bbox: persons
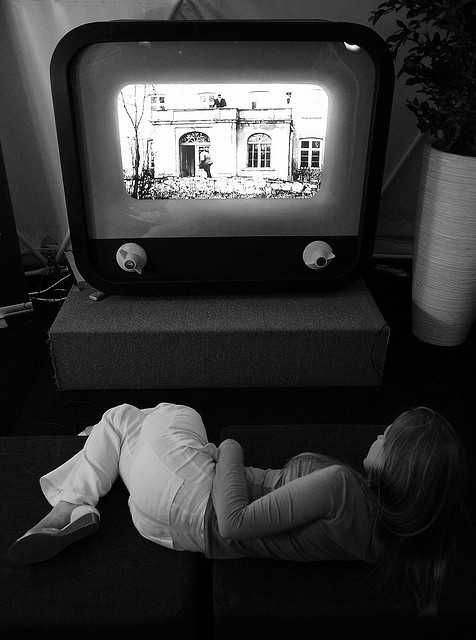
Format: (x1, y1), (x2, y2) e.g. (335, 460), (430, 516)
(6, 400), (459, 565)
(212, 94), (226, 107)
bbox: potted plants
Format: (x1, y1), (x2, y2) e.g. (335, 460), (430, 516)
(368, 0), (476, 346)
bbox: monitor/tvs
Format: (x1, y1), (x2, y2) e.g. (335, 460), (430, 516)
(48, 17), (397, 298)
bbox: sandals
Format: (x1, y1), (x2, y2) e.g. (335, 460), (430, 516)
(7, 505), (101, 564)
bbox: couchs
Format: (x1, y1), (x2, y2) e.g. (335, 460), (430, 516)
(0, 425), (476, 638)
